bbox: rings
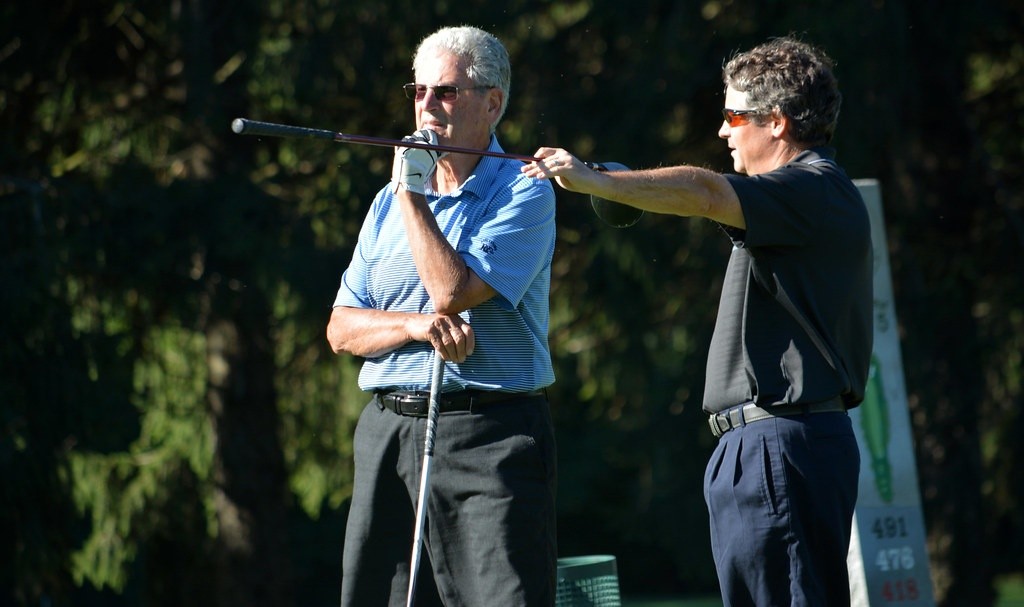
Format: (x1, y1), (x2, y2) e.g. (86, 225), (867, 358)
(553, 160), (559, 166)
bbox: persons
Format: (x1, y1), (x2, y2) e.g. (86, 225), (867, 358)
(327, 24), (560, 607)
(520, 38), (874, 607)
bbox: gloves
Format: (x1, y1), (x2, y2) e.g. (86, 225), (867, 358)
(390, 129), (448, 195)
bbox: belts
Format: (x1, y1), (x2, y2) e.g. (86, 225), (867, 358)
(372, 385), (544, 418)
(709, 394), (845, 436)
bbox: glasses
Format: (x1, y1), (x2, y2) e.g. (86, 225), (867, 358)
(722, 106), (772, 125)
(403, 82), (496, 103)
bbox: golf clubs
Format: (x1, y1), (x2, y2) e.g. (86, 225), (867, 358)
(405, 346), (445, 607)
(229, 118), (643, 229)
